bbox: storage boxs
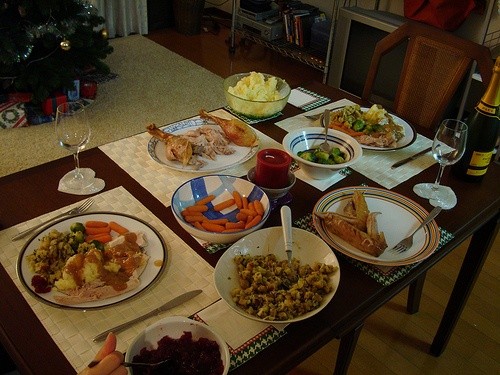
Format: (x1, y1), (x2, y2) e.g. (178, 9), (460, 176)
(311, 21), (337, 53)
(0, 76), (97, 130)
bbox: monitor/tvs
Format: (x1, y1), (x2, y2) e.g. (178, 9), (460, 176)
(328, 6), (410, 111)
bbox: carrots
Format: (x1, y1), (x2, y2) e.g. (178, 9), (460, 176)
(85, 220), (129, 243)
(182, 191), (263, 233)
(328, 120), (366, 137)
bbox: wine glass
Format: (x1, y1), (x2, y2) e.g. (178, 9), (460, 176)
(420, 118), (468, 202)
(55, 102), (94, 190)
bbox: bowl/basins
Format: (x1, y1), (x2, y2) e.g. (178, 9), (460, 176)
(247, 167), (296, 199)
(222, 73), (292, 118)
(123, 316), (230, 375)
(282, 127), (363, 181)
(212, 228), (341, 325)
(170, 174), (271, 244)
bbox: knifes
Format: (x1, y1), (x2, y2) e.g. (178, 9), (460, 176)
(91, 289), (203, 342)
(391, 143), (440, 169)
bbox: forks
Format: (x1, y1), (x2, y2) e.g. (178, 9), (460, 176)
(302, 105), (345, 120)
(11, 196), (95, 242)
(392, 206), (442, 255)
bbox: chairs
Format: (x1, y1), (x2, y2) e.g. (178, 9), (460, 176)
(362, 20), (493, 315)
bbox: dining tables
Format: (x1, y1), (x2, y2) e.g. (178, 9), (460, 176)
(0, 80), (500, 375)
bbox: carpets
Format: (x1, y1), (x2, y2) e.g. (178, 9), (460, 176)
(0, 34), (226, 178)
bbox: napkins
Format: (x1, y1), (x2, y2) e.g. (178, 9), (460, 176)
(197, 299), (272, 350)
(287, 88), (319, 108)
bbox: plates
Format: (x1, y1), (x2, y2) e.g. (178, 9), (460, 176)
(320, 108), (418, 152)
(16, 212), (168, 309)
(147, 117), (261, 173)
(312, 187), (440, 267)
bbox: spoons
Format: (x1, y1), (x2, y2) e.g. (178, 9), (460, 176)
(319, 109), (330, 153)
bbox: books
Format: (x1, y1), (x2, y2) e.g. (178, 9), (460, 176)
(283, 9), (314, 48)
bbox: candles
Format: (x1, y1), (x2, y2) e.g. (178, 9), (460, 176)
(255, 149), (292, 189)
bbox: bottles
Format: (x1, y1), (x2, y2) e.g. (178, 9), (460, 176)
(450, 54), (500, 186)
